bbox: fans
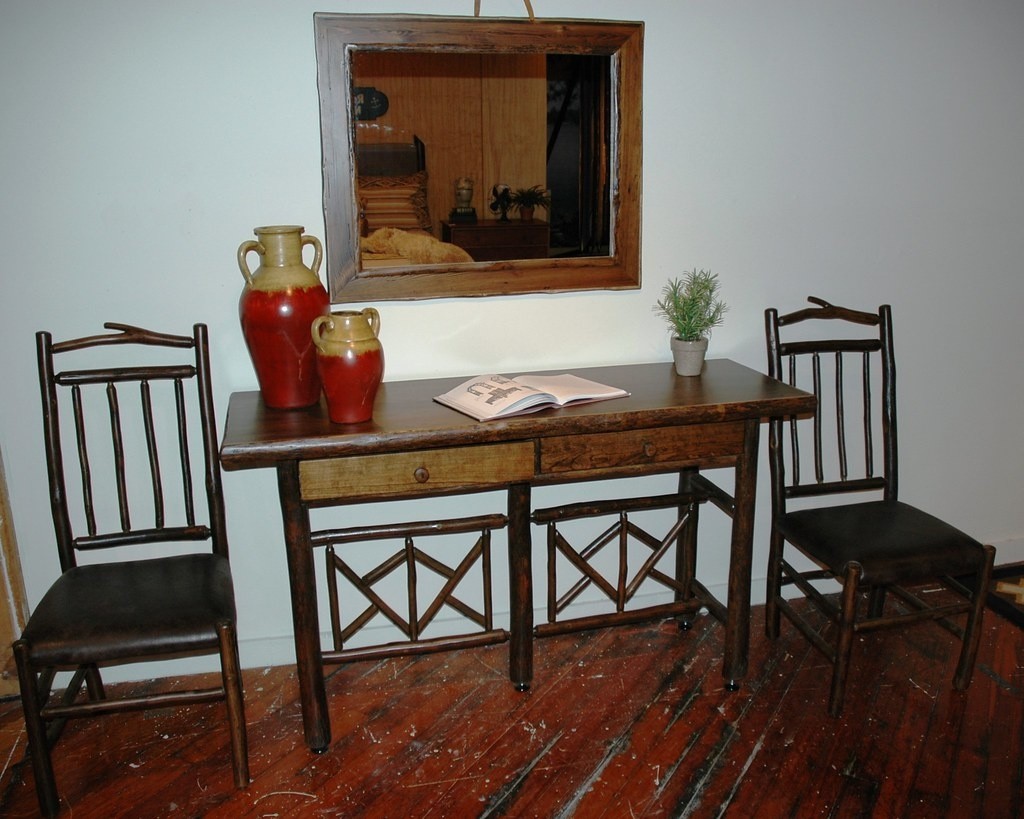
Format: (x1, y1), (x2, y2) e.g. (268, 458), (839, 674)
(486, 183), (513, 223)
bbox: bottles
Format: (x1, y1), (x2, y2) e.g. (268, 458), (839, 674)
(311, 308), (383, 423)
(237, 225), (328, 408)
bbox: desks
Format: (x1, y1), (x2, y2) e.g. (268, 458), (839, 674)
(220, 359), (817, 754)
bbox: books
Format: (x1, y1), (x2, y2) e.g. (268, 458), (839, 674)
(432, 373), (630, 423)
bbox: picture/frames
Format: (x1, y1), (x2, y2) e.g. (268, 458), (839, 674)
(314, 13), (646, 305)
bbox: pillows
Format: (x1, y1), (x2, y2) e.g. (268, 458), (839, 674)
(354, 172), (432, 233)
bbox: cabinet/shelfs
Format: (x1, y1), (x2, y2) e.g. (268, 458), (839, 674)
(441, 218), (549, 264)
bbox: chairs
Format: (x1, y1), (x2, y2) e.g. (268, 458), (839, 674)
(764, 296), (996, 719)
(12, 322), (250, 819)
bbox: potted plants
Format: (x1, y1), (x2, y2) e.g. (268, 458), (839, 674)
(652, 268), (732, 377)
(509, 184), (551, 222)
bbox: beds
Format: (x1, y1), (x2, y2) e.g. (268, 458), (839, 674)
(352, 134), (476, 271)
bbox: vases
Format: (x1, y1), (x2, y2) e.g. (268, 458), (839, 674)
(237, 225), (330, 410)
(311, 307), (385, 424)
